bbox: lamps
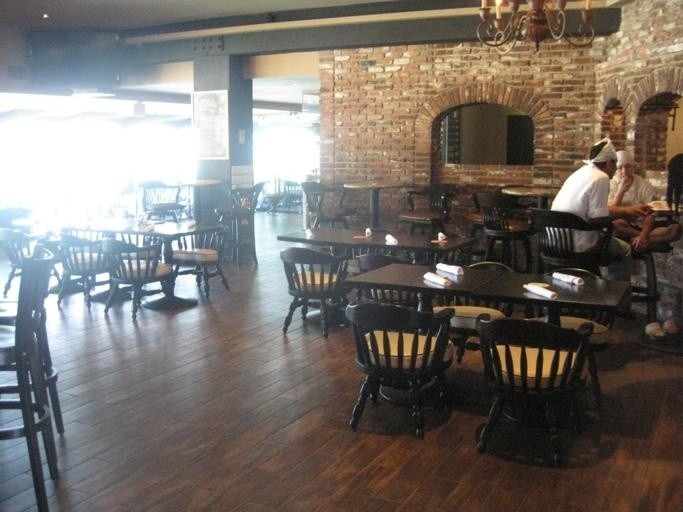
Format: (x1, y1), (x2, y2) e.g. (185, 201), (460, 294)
(473, 1), (598, 56)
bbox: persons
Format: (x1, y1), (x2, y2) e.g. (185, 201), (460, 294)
(667, 152), (682, 215)
(546, 138), (637, 320)
(606, 150), (682, 252)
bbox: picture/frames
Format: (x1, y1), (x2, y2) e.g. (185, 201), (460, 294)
(190, 89), (230, 162)
